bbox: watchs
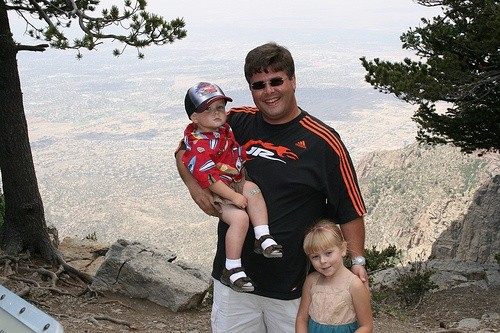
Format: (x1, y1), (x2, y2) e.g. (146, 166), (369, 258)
(350, 256), (365, 265)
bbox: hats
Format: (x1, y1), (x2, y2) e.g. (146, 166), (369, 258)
(184, 81), (232, 118)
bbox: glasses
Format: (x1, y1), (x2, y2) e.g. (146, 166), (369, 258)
(250, 75), (290, 89)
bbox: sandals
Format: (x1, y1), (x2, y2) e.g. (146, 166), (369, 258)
(220, 266), (255, 292)
(253, 234), (283, 258)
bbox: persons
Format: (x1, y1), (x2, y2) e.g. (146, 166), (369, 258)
(176, 81), (283, 292)
(294, 222), (375, 333)
(206, 43), (369, 333)
